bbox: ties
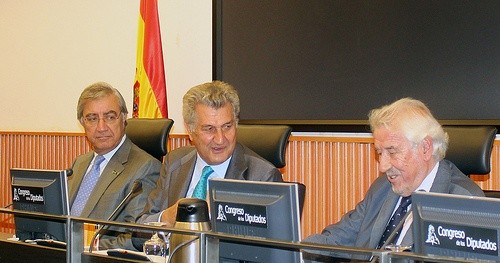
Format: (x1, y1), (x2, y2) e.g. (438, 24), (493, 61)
(192, 166), (214, 201)
(70, 156), (105, 217)
(368, 196), (412, 263)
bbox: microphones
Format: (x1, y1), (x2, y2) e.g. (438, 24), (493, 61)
(88, 180), (142, 254)
(4, 168), (73, 208)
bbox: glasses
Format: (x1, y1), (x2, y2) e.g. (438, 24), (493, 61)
(82, 110), (122, 125)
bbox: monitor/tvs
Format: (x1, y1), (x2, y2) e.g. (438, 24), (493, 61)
(9, 168), (70, 244)
(411, 190), (500, 263)
(208, 179), (303, 263)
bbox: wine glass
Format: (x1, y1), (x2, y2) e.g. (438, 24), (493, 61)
(385, 245), (412, 252)
(143, 221), (167, 263)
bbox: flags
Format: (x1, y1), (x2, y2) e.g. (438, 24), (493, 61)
(135, 0), (169, 120)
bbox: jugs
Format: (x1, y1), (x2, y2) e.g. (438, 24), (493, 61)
(169, 197), (212, 263)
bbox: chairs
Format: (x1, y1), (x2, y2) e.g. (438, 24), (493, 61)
(125, 118), (174, 164)
(442, 125), (500, 198)
(236, 125), (306, 221)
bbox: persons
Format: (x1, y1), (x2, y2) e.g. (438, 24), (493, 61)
(131, 81), (284, 253)
(301, 98), (487, 263)
(64, 81), (160, 250)
